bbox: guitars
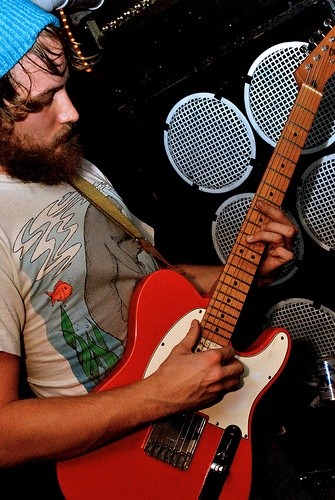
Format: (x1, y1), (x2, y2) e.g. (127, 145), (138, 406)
(55, 17), (335, 500)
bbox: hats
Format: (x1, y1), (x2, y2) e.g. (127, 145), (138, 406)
(0, 0), (62, 78)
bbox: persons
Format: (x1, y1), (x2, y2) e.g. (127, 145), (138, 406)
(0, 0), (300, 469)
